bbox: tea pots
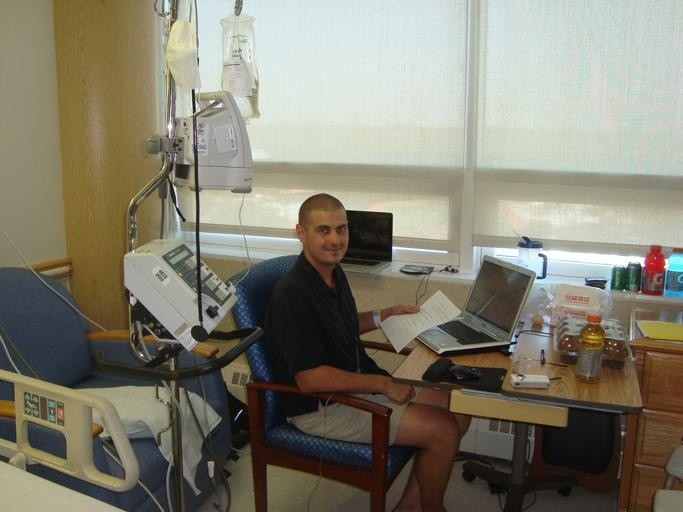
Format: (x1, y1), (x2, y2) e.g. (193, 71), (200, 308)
(515, 236), (547, 281)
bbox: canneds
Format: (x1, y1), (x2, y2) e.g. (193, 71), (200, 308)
(626, 263), (641, 292)
(611, 265), (625, 292)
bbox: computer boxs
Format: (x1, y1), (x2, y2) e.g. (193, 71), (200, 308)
(457, 415), (537, 461)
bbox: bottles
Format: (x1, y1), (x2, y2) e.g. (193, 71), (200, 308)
(665, 247), (683, 298)
(641, 245), (665, 295)
(575, 311), (605, 386)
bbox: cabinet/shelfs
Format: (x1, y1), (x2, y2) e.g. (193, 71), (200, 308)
(616, 334), (682, 511)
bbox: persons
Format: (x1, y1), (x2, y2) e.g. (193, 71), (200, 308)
(262, 193), (471, 511)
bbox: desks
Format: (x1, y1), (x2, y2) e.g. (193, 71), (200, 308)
(390, 320), (644, 512)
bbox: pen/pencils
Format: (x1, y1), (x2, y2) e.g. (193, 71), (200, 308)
(541, 349), (545, 365)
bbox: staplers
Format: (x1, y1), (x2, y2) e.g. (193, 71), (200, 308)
(585, 277), (608, 289)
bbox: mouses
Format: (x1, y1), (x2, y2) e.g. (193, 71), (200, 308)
(450, 364), (481, 382)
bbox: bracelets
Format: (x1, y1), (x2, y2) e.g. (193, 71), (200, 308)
(371, 308), (384, 330)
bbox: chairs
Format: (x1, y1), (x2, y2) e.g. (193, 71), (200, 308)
(0, 266), (235, 511)
(224, 253), (418, 512)
(650, 443), (682, 512)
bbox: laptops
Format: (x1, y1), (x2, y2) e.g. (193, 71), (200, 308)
(339, 209), (393, 274)
(414, 255), (536, 356)
